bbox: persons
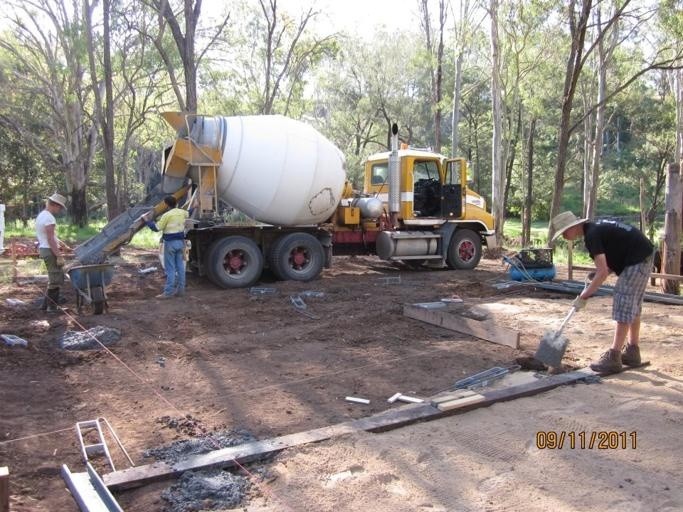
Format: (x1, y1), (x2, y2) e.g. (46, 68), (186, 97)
(34, 192), (75, 315)
(550, 209), (655, 374)
(141, 195), (189, 302)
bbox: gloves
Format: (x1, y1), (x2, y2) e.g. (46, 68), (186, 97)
(63, 245), (72, 254)
(572, 296), (587, 312)
(57, 255), (65, 268)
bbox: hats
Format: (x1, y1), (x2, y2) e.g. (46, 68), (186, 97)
(46, 193), (67, 211)
(549, 211), (590, 242)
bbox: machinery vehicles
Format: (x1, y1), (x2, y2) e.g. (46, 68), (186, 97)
(70, 109), (499, 289)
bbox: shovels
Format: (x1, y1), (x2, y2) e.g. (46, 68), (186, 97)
(534, 287), (584, 368)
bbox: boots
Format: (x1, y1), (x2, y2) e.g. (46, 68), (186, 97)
(39, 286), (70, 313)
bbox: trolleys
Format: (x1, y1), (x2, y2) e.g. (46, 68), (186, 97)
(66, 263), (115, 316)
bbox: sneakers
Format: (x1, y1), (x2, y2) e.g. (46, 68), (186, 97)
(155, 293), (185, 300)
(590, 349), (622, 372)
(620, 344), (641, 364)
